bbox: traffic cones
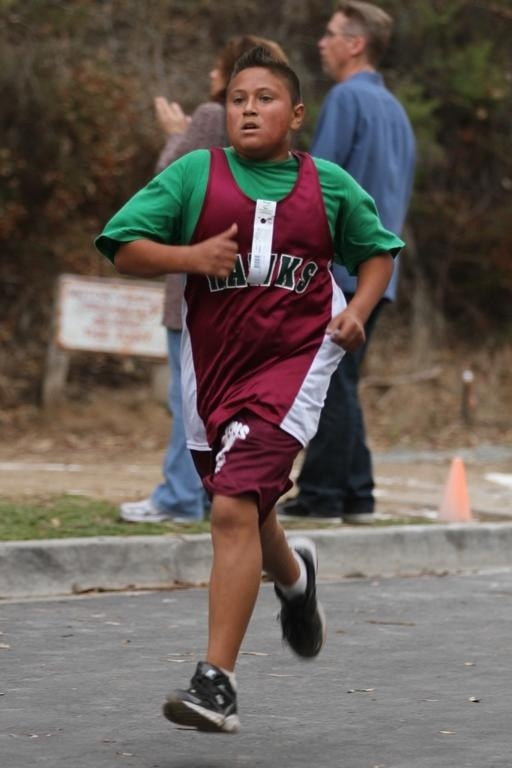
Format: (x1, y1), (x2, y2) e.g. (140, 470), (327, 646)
(436, 455), (475, 523)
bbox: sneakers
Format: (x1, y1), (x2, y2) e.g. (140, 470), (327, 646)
(121, 494), (203, 528)
(275, 493), (376, 525)
(273, 535), (327, 659)
(163, 660), (242, 734)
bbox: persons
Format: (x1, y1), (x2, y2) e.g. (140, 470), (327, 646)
(276, 1), (417, 512)
(94, 44), (406, 732)
(119, 31), (290, 527)
